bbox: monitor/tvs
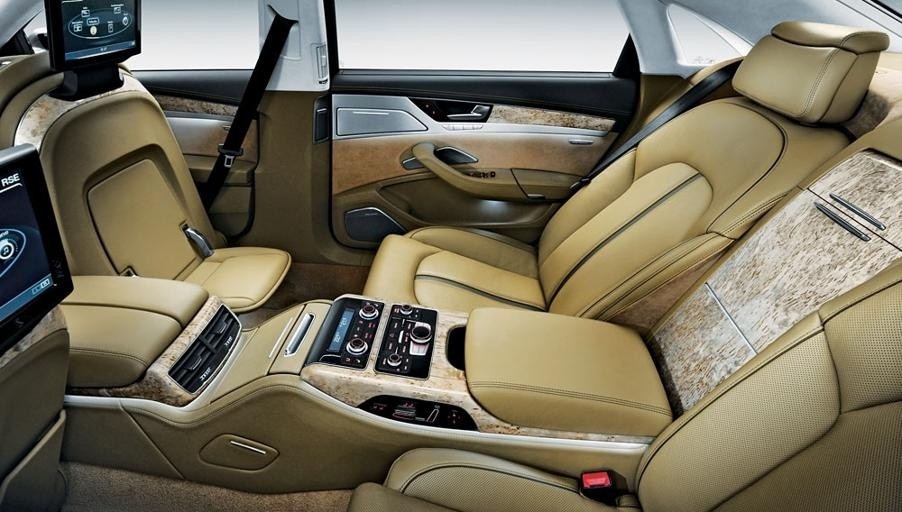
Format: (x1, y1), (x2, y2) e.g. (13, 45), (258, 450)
(0, 142), (73, 357)
(44, 0), (144, 102)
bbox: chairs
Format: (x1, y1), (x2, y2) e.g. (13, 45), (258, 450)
(0, 306), (70, 512)
(345, 256), (902, 512)
(0, 50), (292, 311)
(359, 17), (889, 323)
